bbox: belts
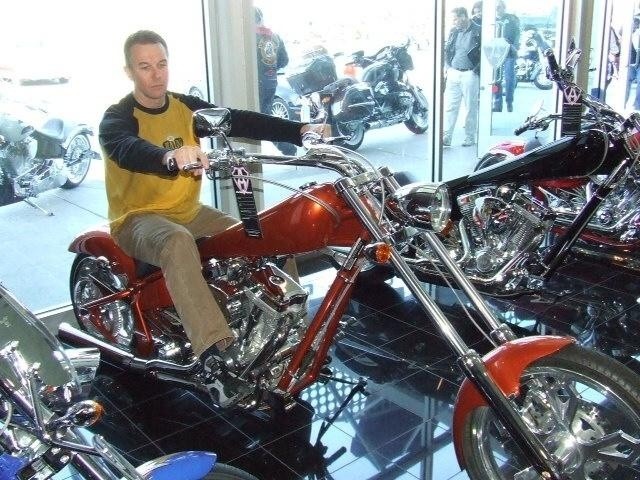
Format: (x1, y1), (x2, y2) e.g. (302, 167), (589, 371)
(452, 67), (473, 71)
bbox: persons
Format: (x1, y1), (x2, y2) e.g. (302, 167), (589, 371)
(442, 1), (519, 147)
(98, 29), (332, 410)
(256, 8), (296, 156)
(604, 18), (640, 112)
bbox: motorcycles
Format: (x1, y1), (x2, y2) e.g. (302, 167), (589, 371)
(321, 33), (640, 300)
(325, 275), (640, 461)
(493, 26), (554, 96)
(546, 39), (616, 92)
(64, 359), (366, 480)
(0, 112), (102, 209)
(473, 99), (639, 273)
(0, 284), (256, 479)
(285, 29), (429, 154)
(57, 82), (639, 479)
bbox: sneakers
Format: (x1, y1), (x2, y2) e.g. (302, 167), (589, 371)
(205, 360), (255, 408)
(493, 107), (502, 112)
(462, 136), (475, 146)
(507, 103), (513, 113)
(443, 136), (451, 147)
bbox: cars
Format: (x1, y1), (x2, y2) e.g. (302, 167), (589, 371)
(181, 42), (357, 122)
(501, 17), (622, 78)
